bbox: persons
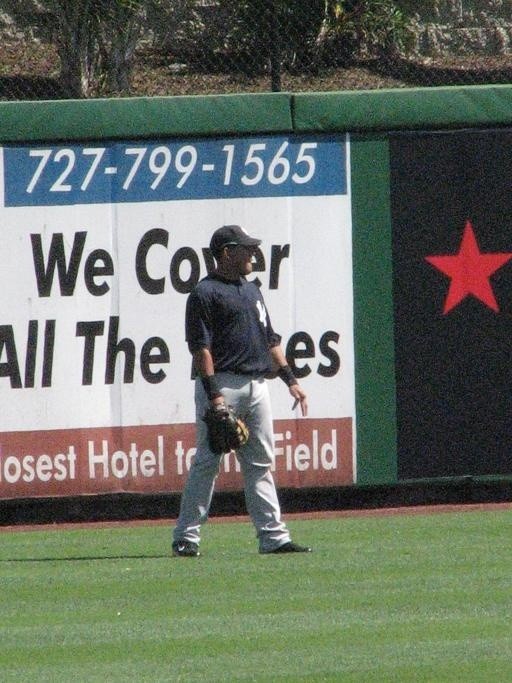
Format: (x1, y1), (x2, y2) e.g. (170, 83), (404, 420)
(171, 224), (314, 557)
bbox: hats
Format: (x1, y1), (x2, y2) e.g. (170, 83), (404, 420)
(210, 226), (262, 252)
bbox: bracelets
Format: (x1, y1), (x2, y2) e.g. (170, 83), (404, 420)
(200, 374), (223, 401)
(276, 364), (298, 387)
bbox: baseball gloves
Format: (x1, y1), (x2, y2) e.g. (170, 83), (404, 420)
(201, 403), (249, 455)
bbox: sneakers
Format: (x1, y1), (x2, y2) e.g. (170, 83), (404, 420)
(172, 540), (200, 556)
(274, 543), (313, 553)
